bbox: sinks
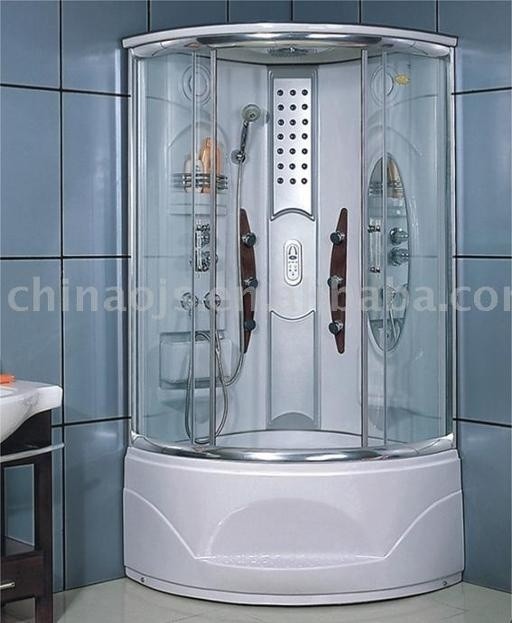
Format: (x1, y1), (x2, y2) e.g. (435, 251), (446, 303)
(0, 376), (62, 442)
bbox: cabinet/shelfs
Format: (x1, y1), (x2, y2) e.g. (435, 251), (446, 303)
(1, 410), (52, 622)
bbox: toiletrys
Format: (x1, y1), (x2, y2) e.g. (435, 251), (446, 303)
(202, 138), (220, 193)
(185, 152), (203, 191)
